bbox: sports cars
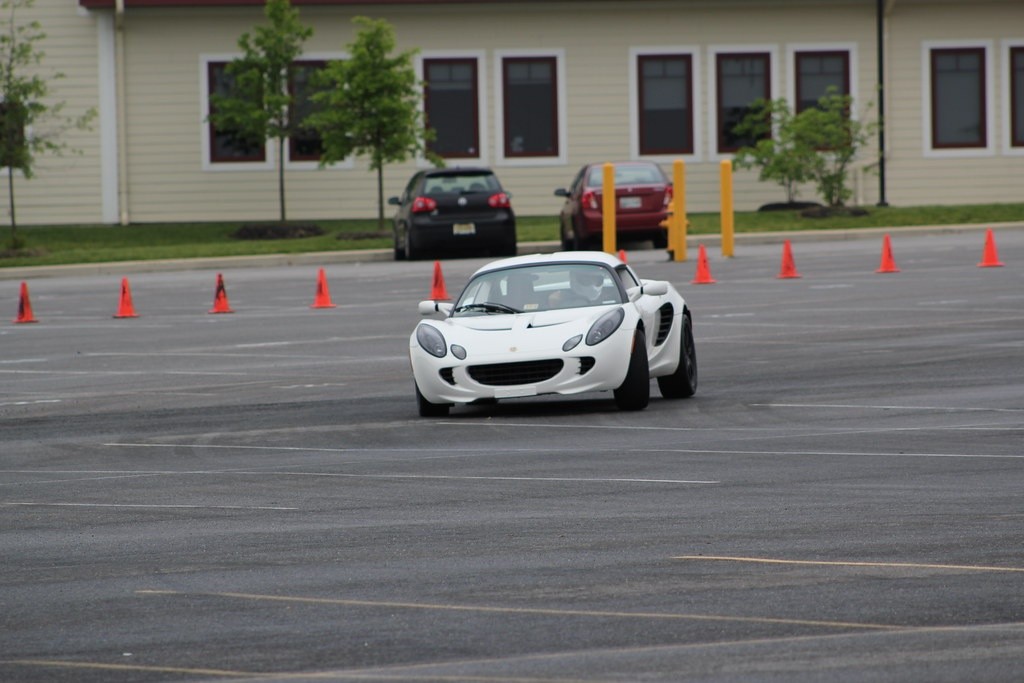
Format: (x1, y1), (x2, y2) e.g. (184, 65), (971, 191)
(407, 248), (699, 420)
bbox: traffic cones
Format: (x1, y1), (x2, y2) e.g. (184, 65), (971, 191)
(427, 261), (452, 301)
(688, 241), (719, 285)
(776, 238), (800, 279)
(977, 228), (1007, 267)
(309, 267), (337, 308)
(111, 277), (142, 318)
(11, 281), (40, 323)
(208, 273), (237, 314)
(874, 233), (903, 273)
(618, 250), (627, 264)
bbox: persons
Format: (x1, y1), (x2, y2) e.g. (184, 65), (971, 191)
(548, 267), (615, 308)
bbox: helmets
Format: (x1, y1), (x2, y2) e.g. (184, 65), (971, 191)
(570, 266), (605, 301)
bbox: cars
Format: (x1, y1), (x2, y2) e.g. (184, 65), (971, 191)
(387, 163), (519, 260)
(553, 161), (674, 250)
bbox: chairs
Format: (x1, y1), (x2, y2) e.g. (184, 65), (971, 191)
(505, 273), (538, 310)
(429, 186), (443, 195)
(469, 183), (486, 192)
